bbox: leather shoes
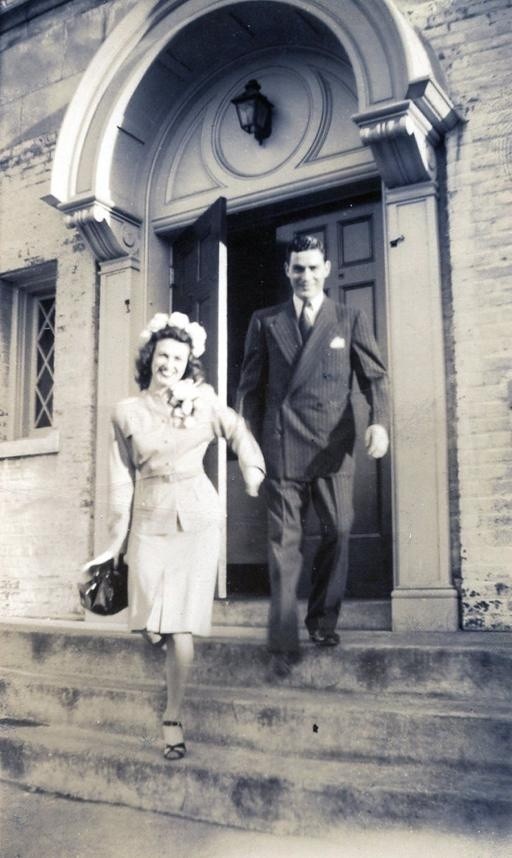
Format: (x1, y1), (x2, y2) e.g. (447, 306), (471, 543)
(309, 628), (340, 646)
(270, 650), (300, 678)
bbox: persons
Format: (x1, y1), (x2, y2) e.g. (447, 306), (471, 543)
(79, 309), (268, 763)
(232, 231), (395, 686)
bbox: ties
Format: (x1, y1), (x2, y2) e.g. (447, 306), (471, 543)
(299, 297), (313, 345)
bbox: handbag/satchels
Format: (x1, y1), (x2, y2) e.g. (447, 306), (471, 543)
(75, 555), (127, 616)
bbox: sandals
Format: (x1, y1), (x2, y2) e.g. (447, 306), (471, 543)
(162, 721), (186, 760)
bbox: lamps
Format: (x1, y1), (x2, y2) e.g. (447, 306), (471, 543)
(231, 79), (274, 145)
